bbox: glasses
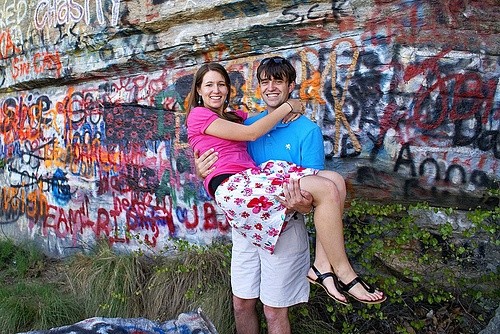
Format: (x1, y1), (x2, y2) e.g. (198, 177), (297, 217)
(260, 56), (291, 65)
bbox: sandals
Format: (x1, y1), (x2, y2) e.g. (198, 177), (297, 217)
(305, 264), (352, 306)
(337, 275), (388, 304)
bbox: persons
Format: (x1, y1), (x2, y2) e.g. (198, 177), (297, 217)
(193, 56), (326, 334)
(184, 63), (388, 307)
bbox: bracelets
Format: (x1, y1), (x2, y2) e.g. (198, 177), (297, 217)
(301, 205), (313, 216)
(285, 100), (293, 111)
